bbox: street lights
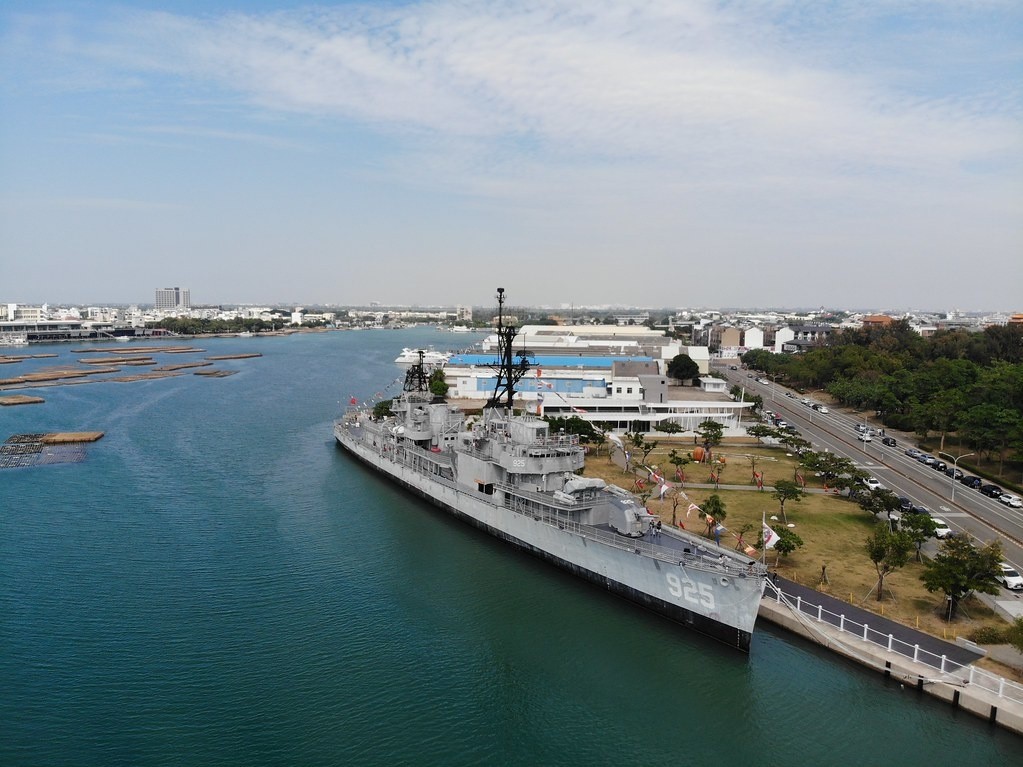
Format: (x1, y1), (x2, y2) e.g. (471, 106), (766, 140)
(801, 388), (823, 421)
(939, 452), (975, 501)
(852, 410), (881, 452)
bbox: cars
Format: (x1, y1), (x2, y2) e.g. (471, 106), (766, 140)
(747, 374), (753, 378)
(931, 461), (947, 471)
(979, 484), (1003, 498)
(998, 494), (1022, 508)
(918, 454), (936, 464)
(731, 366), (737, 370)
(944, 468), (963, 479)
(755, 377), (769, 385)
(992, 563), (1023, 589)
(961, 476), (982, 489)
(858, 433), (872, 442)
(785, 392), (828, 414)
(905, 449), (921, 458)
(852, 471), (913, 513)
(882, 437), (896, 447)
(930, 519), (952, 539)
(864, 427), (875, 436)
(911, 507), (931, 517)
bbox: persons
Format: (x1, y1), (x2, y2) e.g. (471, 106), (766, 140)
(719, 554), (723, 559)
(656, 521), (662, 537)
(773, 570), (777, 586)
(649, 520), (655, 536)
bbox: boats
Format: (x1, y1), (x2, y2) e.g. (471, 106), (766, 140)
(239, 331), (253, 338)
(395, 347), (447, 364)
(0, 336), (29, 345)
(326, 329), (768, 656)
(116, 336), (129, 340)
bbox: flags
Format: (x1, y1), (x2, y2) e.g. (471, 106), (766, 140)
(536, 370), (761, 559)
(351, 375), (403, 412)
(762, 523), (780, 550)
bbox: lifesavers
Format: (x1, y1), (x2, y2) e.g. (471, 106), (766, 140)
(431, 447), (441, 452)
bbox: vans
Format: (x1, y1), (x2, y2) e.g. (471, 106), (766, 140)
(854, 423), (866, 432)
